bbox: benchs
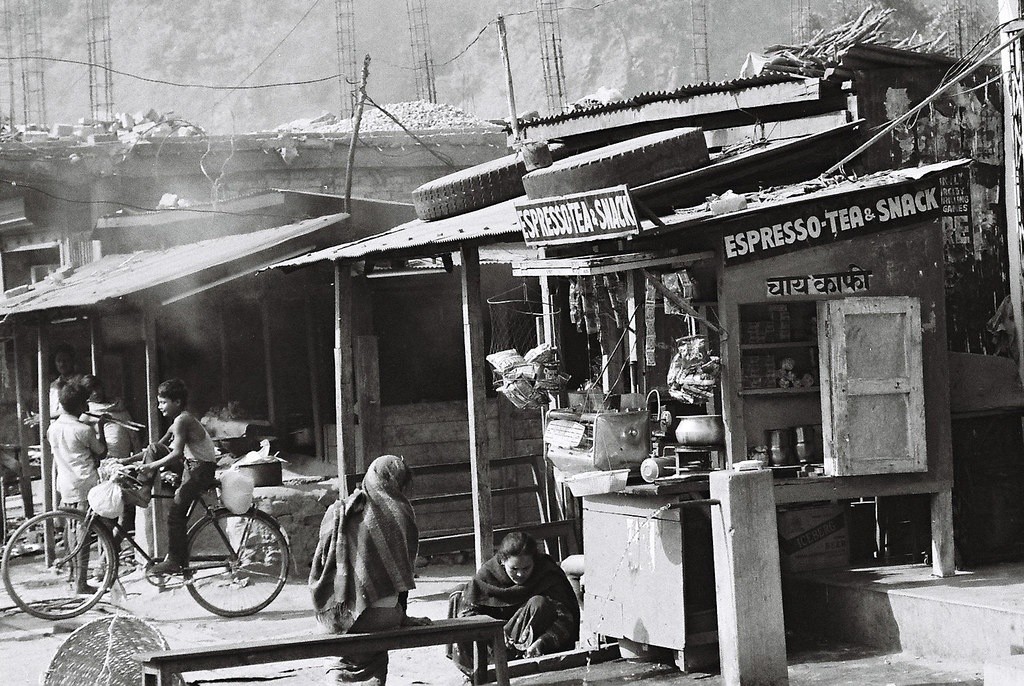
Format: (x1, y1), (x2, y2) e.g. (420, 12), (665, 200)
(130, 615), (508, 686)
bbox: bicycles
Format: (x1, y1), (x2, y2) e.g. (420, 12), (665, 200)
(1, 450), (290, 621)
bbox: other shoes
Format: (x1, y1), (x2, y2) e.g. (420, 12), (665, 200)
(122, 489), (148, 509)
(152, 560), (182, 574)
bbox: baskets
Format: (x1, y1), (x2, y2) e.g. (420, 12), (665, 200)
(42, 615), (185, 686)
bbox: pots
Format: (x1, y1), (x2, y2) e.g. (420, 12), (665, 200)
(239, 461), (283, 487)
(223, 436), (281, 458)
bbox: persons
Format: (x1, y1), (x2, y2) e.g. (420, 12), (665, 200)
(107, 378), (216, 573)
(47, 382), (112, 594)
(308, 455), (420, 686)
(446, 532), (581, 686)
(23, 375), (144, 578)
(49, 344), (78, 425)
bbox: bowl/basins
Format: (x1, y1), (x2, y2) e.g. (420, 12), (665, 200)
(675, 414), (725, 446)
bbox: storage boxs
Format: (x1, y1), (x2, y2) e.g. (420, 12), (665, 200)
(780, 505), (851, 573)
